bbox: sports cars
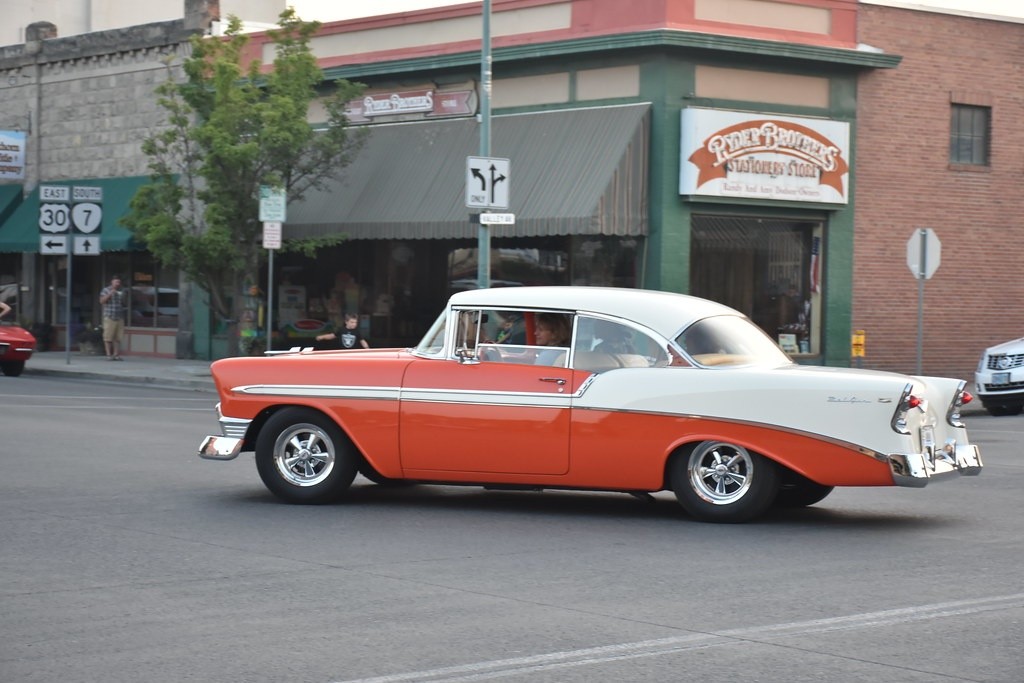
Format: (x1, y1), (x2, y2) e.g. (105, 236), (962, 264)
(195, 285), (986, 523)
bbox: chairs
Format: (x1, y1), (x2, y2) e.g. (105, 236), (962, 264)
(552, 351), (650, 370)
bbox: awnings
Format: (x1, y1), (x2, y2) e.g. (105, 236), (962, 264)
(256, 102), (649, 235)
(0, 174), (181, 253)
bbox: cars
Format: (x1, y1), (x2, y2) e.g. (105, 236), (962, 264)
(973, 336), (1024, 418)
(0, 320), (37, 378)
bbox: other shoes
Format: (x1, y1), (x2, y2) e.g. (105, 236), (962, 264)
(113, 355), (123, 361)
(106, 356), (113, 360)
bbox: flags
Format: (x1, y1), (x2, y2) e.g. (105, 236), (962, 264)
(810, 236), (821, 293)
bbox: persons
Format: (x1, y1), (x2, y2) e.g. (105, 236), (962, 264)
(316, 312), (370, 349)
(497, 311), (635, 367)
(0, 301), (12, 319)
(99, 274), (128, 361)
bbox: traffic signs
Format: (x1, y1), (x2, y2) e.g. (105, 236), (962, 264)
(40, 185), (71, 255)
(72, 186), (103, 257)
